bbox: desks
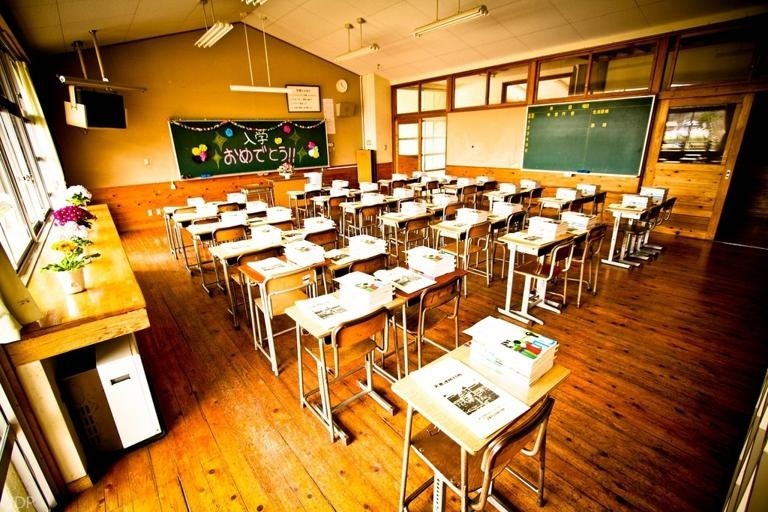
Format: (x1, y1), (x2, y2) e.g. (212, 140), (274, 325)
(392, 340), (572, 512)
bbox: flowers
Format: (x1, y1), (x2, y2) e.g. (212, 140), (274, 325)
(53, 206), (96, 230)
(40, 240), (101, 273)
(62, 185), (93, 207)
(277, 163), (295, 173)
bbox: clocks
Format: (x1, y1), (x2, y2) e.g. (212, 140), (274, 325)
(336, 79), (348, 94)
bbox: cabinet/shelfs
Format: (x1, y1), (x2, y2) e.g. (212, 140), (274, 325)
(0, 203), (151, 477)
(722, 356), (766, 511)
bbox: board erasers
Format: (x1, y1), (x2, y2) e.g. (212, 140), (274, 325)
(577, 169), (591, 174)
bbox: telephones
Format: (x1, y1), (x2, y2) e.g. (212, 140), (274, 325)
(168, 120), (330, 181)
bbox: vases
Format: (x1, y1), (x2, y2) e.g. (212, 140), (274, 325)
(65, 221), (88, 239)
(65, 235), (100, 265)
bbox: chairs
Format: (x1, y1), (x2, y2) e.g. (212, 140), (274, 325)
(164, 170), (678, 444)
(402, 393), (555, 512)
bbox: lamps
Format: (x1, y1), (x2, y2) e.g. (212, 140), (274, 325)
(55, 30), (147, 93)
(334, 17), (380, 62)
(412, 0), (488, 37)
(230, 12), (293, 94)
(194, 0), (234, 48)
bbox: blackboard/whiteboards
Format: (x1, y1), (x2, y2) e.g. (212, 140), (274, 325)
(521, 95), (656, 178)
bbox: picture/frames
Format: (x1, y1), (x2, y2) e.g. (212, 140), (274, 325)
(285, 84), (321, 113)
(64, 267), (85, 294)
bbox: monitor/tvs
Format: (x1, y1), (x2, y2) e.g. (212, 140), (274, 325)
(75, 88), (125, 128)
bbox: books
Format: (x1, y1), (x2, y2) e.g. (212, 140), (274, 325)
(186, 171), (669, 440)
